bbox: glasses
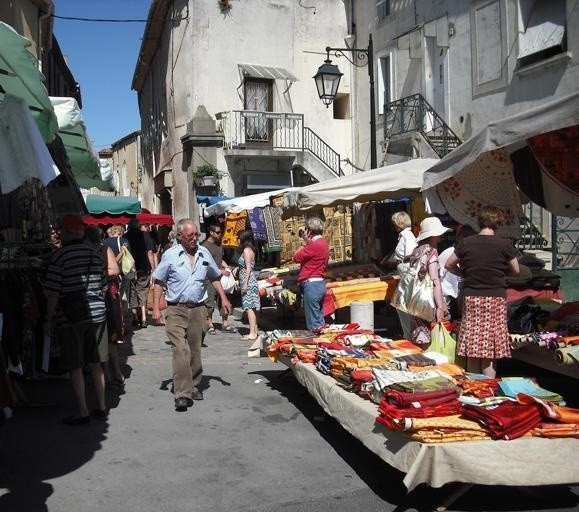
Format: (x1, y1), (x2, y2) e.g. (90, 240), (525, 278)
(180, 233), (201, 239)
(212, 231), (221, 235)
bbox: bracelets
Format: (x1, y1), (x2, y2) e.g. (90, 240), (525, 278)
(302, 244), (307, 248)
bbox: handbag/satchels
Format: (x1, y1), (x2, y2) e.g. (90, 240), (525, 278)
(147, 284), (167, 311)
(508, 305), (550, 334)
(59, 291), (88, 318)
(390, 248), (437, 321)
(221, 267), (235, 294)
(121, 246), (137, 280)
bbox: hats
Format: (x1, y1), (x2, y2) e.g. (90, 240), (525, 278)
(416, 217), (453, 242)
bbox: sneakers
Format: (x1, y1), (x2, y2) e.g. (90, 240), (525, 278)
(140, 321), (148, 327)
(175, 397), (189, 408)
(192, 391), (203, 400)
(132, 320), (138, 325)
(65, 379), (122, 426)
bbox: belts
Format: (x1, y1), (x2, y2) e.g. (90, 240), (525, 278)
(307, 278), (324, 282)
(168, 301), (205, 308)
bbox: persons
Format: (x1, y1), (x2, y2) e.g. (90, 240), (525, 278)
(386, 203), (521, 378)
(235, 228), (263, 341)
(291, 216), (332, 332)
(4, 210), (238, 426)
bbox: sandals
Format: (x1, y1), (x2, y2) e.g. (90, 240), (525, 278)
(208, 320), (256, 340)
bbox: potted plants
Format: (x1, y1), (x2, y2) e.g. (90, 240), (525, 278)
(193, 164), (222, 188)
